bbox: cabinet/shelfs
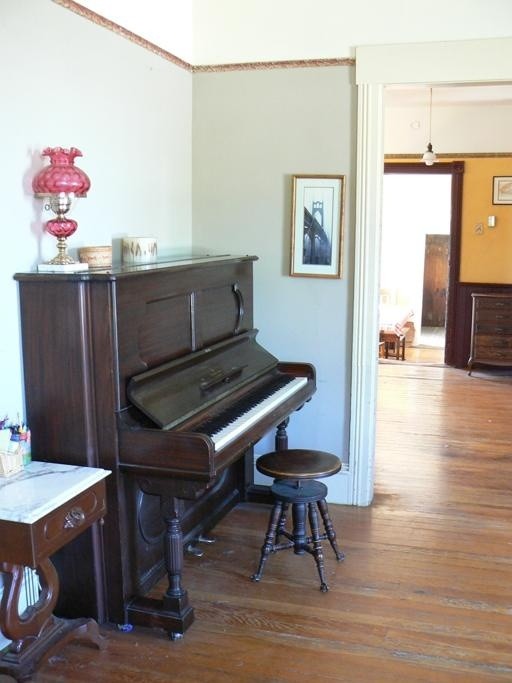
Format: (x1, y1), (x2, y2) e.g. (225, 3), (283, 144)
(467, 292), (512, 376)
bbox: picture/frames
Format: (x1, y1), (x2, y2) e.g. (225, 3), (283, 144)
(289, 175), (345, 280)
(492, 176), (512, 205)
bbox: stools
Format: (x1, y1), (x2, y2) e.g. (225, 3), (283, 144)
(250, 449), (345, 592)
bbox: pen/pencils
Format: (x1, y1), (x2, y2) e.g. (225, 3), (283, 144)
(0, 411), (30, 440)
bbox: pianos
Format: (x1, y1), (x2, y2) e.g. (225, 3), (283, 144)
(16, 252), (314, 638)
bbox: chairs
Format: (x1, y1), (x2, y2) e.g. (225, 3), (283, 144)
(379, 330), (405, 361)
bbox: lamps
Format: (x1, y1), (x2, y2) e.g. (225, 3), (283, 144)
(420, 88), (439, 166)
(32, 146), (90, 272)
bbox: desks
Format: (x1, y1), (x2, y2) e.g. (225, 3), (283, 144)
(0, 460), (112, 683)
